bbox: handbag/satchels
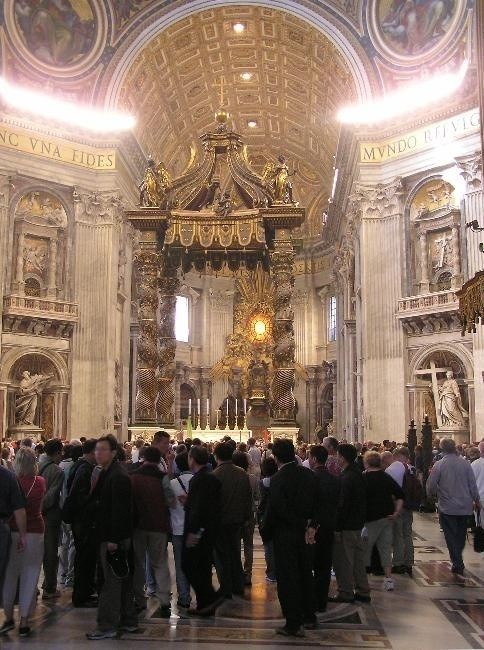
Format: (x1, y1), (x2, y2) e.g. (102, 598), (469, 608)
(471, 526), (484, 553)
(58, 492), (74, 525)
(400, 473), (426, 503)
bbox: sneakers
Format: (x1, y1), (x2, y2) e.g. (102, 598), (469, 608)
(327, 578), (393, 603)
(0, 620), (15, 634)
(451, 564), (464, 574)
(72, 592), (192, 641)
(41, 589), (61, 599)
(17, 624), (32, 637)
(275, 614), (316, 637)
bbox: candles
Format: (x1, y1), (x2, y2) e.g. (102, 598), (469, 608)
(226, 398), (228, 416)
(188, 398), (191, 415)
(206, 398), (209, 414)
(235, 399), (238, 416)
(197, 399), (200, 415)
(243, 398), (246, 414)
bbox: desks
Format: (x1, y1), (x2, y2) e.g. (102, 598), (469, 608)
(182, 428), (254, 446)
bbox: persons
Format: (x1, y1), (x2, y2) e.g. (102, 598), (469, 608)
(438, 370), (468, 425)
(139, 158), (166, 208)
(17, 193), (64, 275)
(0, 423), (482, 643)
(414, 184), (455, 219)
(271, 155), (297, 206)
(15, 368), (52, 424)
